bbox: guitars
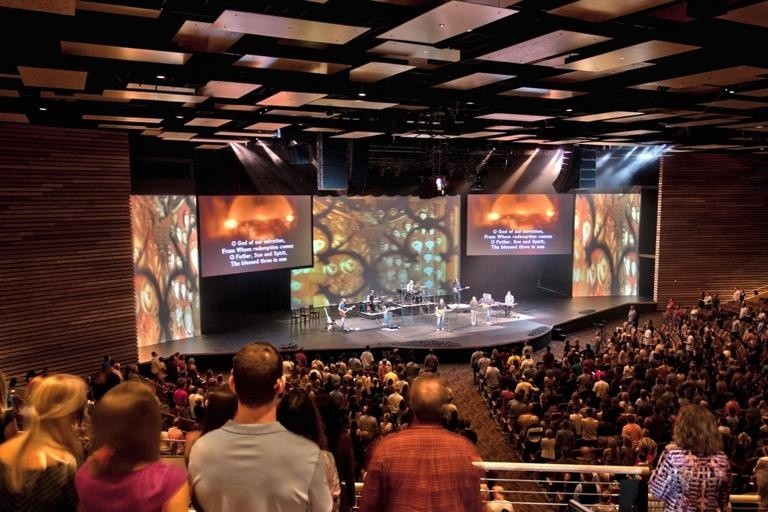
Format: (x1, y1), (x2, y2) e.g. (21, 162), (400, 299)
(434, 309), (454, 318)
(455, 286), (470, 293)
(338, 305), (356, 317)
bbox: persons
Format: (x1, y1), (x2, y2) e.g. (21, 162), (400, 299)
(7, 346), (513, 512)
(505, 290), (514, 316)
(276, 388), (342, 512)
(368, 289), (375, 310)
(0, 374), (88, 511)
(406, 280), (414, 291)
(189, 342), (333, 512)
(648, 404), (731, 512)
(435, 298), (450, 331)
(470, 296), (479, 326)
(75, 382), (190, 511)
(313, 393), (356, 512)
(359, 372), (486, 511)
(471, 287), (767, 512)
(339, 298), (352, 329)
(452, 279), (461, 303)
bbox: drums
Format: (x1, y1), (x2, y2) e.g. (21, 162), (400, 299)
(411, 295), (423, 304)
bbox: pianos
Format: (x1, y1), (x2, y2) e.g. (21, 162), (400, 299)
(496, 301), (519, 308)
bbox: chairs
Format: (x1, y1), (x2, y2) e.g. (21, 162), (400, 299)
(289, 304), (320, 324)
